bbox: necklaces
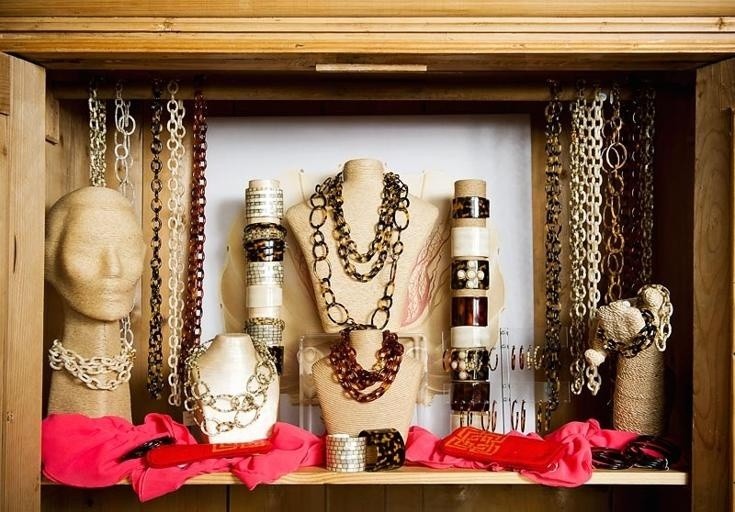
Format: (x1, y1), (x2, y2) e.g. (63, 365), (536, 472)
(330, 327), (404, 402)
(309, 172), (411, 329)
(545, 83), (673, 410)
(89, 84), (209, 408)
(180, 337), (277, 438)
(48, 338), (136, 391)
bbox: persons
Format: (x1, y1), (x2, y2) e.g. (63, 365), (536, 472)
(47, 186), (146, 425)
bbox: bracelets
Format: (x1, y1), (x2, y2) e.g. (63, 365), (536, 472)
(243, 187), (287, 376)
(585, 433), (683, 474)
(443, 196), (497, 432)
(511, 399), (551, 434)
(358, 426), (407, 473)
(512, 345), (542, 370)
(323, 431), (369, 474)
